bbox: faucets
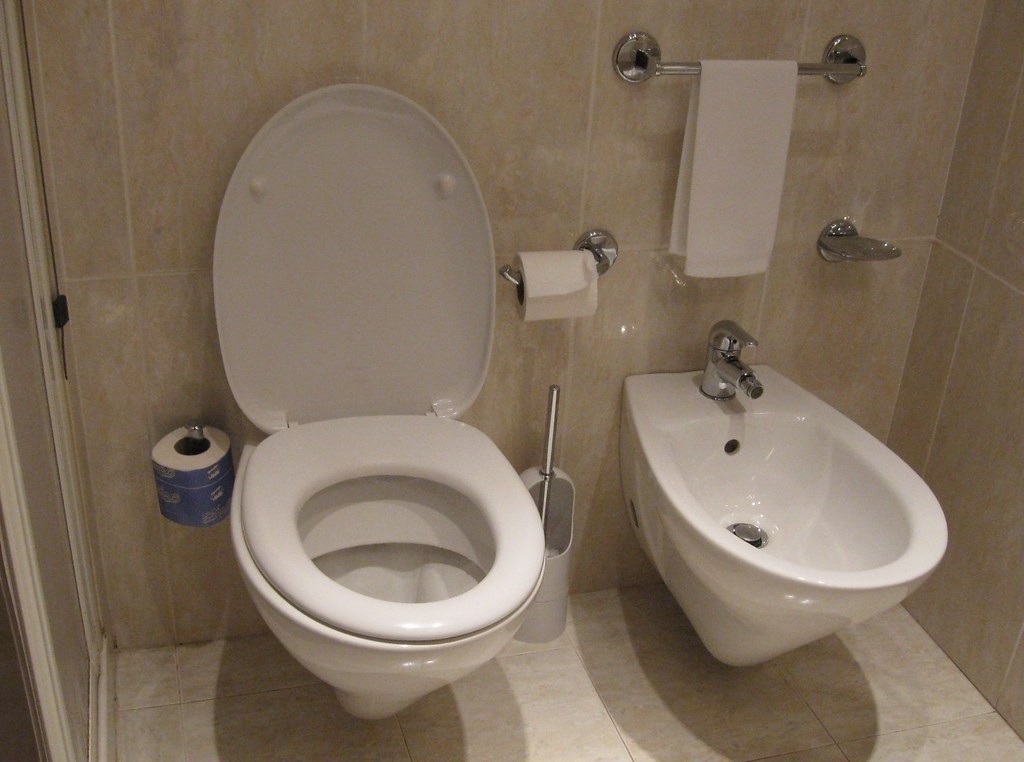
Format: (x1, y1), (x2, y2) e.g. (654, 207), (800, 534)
(698, 317), (765, 405)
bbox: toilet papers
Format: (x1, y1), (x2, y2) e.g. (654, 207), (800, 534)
(513, 248), (600, 325)
(150, 424), (233, 527)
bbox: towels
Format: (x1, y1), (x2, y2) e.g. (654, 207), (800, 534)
(666, 58), (800, 280)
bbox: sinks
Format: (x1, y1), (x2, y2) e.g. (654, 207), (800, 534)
(620, 359), (950, 670)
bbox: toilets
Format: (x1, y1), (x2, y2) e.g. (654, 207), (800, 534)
(210, 82), (547, 722)
